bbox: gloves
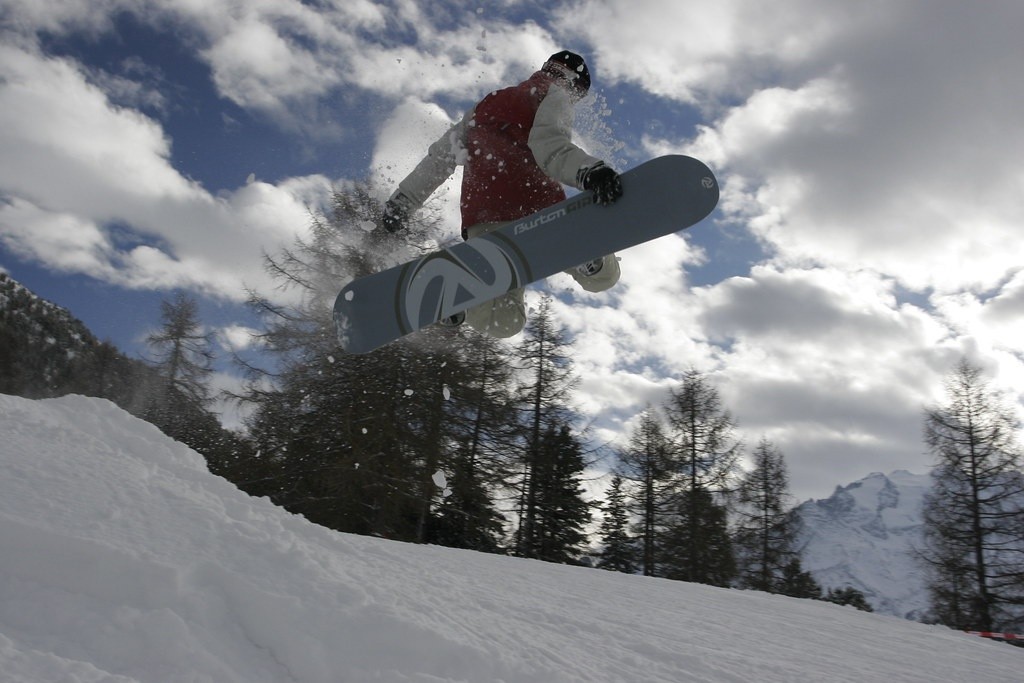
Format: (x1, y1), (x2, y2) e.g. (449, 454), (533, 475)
(382, 200), (409, 234)
(585, 164), (624, 208)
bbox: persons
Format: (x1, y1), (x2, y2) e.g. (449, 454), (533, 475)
(380, 49), (623, 340)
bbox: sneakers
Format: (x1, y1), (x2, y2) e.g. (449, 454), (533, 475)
(439, 309), (468, 328)
(576, 256), (603, 277)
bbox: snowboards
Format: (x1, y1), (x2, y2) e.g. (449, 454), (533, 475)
(332, 153), (722, 356)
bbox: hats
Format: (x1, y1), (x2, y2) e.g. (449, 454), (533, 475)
(541, 50), (591, 99)
